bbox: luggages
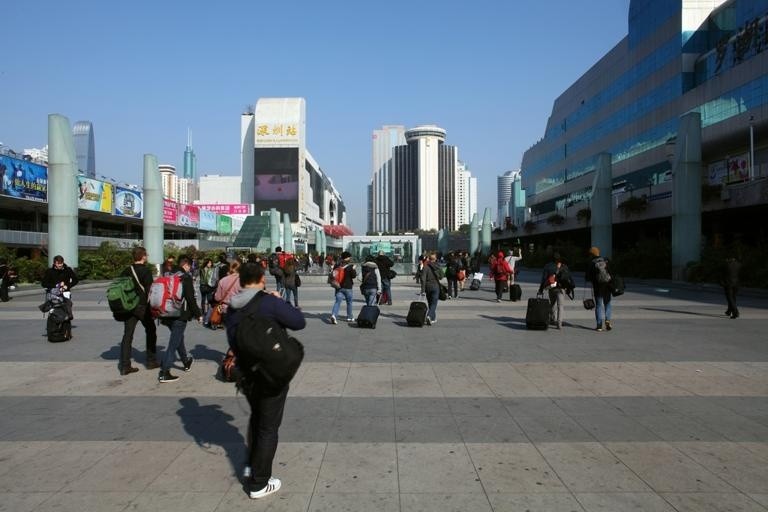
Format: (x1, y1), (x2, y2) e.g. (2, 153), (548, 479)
(470, 272), (483, 290)
(526, 293), (550, 330)
(510, 272), (522, 302)
(407, 292), (427, 327)
(47, 291), (71, 342)
(357, 292), (384, 329)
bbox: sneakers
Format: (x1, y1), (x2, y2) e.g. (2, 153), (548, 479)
(595, 321), (612, 331)
(120, 357), (193, 382)
(243, 466), (282, 499)
(427, 316), (437, 325)
(331, 315), (356, 324)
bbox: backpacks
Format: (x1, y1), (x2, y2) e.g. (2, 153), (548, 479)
(5, 270), (18, 285)
(556, 268), (576, 300)
(445, 262), (458, 278)
(106, 266), (149, 321)
(495, 259), (508, 276)
(224, 292), (305, 397)
(147, 271), (187, 318)
(205, 262), (224, 288)
(592, 257), (611, 282)
(268, 253), (285, 278)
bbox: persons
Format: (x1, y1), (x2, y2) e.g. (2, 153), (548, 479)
(421, 252), (445, 326)
(331, 252), (356, 325)
(159, 255), (203, 383)
(226, 261), (306, 499)
(585, 247), (616, 331)
(538, 253), (569, 329)
(416, 249), (482, 299)
(488, 248), (522, 302)
(272, 247), (300, 308)
(716, 251), (742, 318)
(0, 258), (9, 302)
(361, 250), (395, 306)
(120, 247), (162, 375)
(41, 255), (78, 291)
(293, 252), (334, 273)
(165, 250), (267, 327)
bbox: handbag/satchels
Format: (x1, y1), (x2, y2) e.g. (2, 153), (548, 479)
(457, 272), (464, 281)
(328, 264), (350, 290)
(439, 284), (447, 301)
(222, 346), (237, 382)
(209, 303), (221, 325)
(295, 274), (301, 287)
(611, 275), (625, 297)
(542, 274), (557, 290)
(584, 299), (594, 310)
(386, 270), (397, 279)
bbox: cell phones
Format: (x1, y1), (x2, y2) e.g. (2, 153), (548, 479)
(279, 288), (286, 297)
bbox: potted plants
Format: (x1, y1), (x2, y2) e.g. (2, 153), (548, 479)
(617, 195), (651, 220)
